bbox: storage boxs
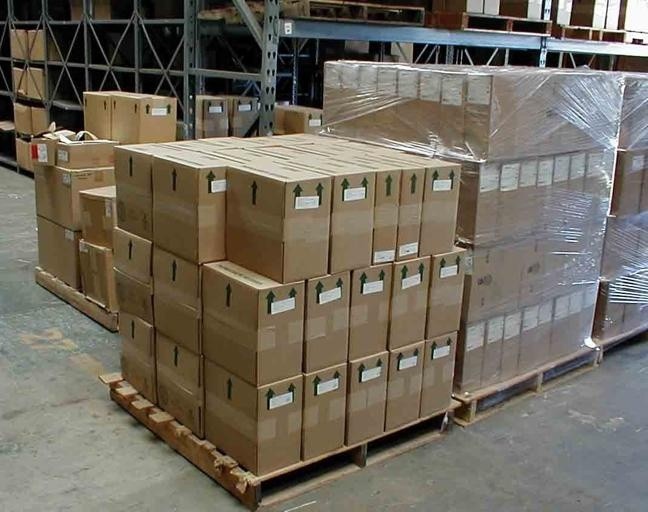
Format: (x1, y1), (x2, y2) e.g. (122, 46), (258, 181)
(11, 28), (52, 171)
